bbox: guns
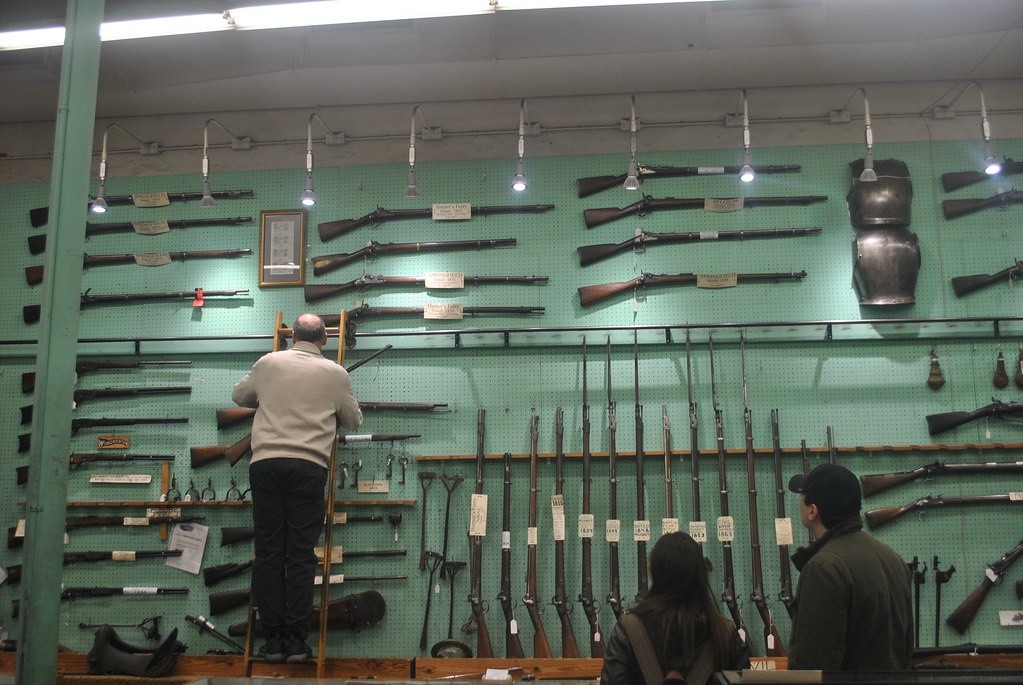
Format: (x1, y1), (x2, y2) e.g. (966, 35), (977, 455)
(0, 156), (1023, 658)
(220, 342), (395, 469)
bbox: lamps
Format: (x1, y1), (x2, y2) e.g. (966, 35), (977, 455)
(302, 112), (346, 205)
(935, 81), (1001, 175)
(620, 94), (640, 191)
(202, 118), (251, 207)
(725, 87), (756, 183)
(512, 98), (542, 192)
(404, 105), (442, 199)
(91, 123), (159, 215)
(828, 88), (877, 181)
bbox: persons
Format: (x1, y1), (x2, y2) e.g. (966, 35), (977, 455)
(788, 464), (916, 685)
(600, 532), (749, 685)
(231, 313), (364, 662)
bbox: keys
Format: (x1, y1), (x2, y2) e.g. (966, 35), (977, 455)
(337, 462), (348, 489)
(398, 457), (408, 484)
(350, 463), (363, 488)
(386, 454), (395, 478)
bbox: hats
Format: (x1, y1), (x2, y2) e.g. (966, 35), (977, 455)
(788, 463), (862, 512)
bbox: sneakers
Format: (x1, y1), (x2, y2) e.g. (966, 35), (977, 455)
(284, 638), (311, 663)
(259, 641), (283, 662)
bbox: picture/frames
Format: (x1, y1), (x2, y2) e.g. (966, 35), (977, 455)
(258, 208), (304, 286)
